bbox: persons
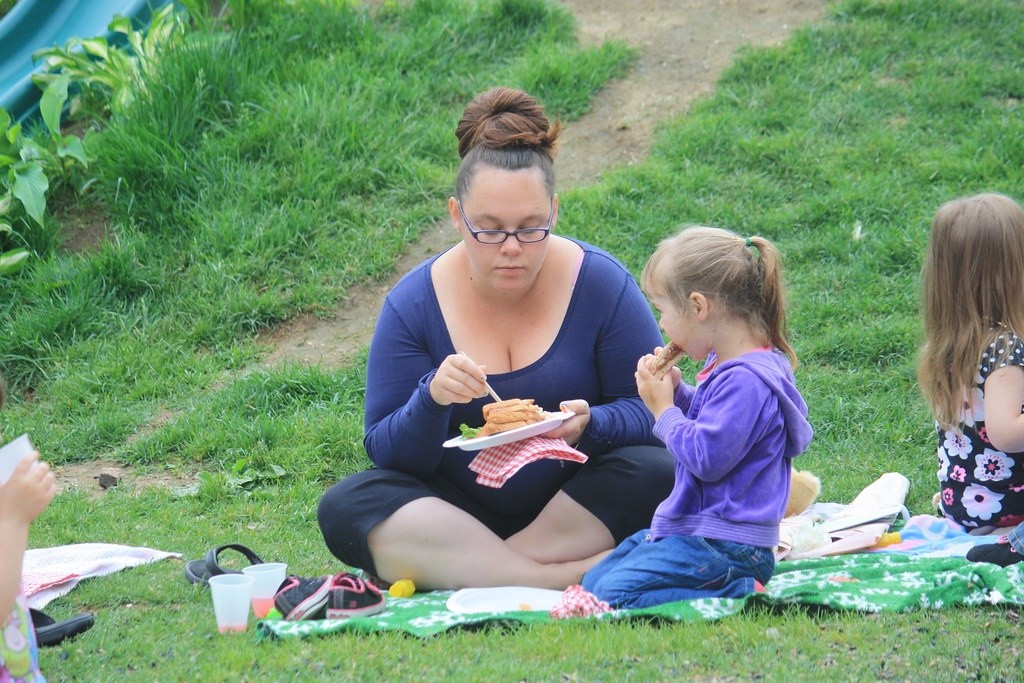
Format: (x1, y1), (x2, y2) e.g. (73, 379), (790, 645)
(318, 88), (676, 592)
(919, 194), (1024, 565)
(582, 224), (812, 610)
(0, 448), (59, 683)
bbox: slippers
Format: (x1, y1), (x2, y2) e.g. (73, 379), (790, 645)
(30, 607), (95, 648)
(185, 544), (266, 586)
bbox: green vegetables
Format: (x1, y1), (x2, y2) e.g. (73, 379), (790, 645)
(459, 424), (481, 438)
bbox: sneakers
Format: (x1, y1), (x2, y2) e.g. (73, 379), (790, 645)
(325, 573), (384, 620)
(272, 575), (333, 620)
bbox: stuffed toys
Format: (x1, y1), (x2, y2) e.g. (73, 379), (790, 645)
(779, 467), (832, 556)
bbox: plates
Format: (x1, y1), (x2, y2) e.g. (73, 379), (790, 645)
(442, 410), (576, 452)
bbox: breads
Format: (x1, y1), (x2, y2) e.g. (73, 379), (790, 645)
(651, 342), (685, 379)
(475, 397), (544, 436)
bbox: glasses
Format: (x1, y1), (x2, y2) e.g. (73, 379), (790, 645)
(458, 197), (554, 244)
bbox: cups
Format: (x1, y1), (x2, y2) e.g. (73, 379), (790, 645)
(207, 573), (253, 636)
(0, 433), (40, 485)
(240, 562), (287, 621)
